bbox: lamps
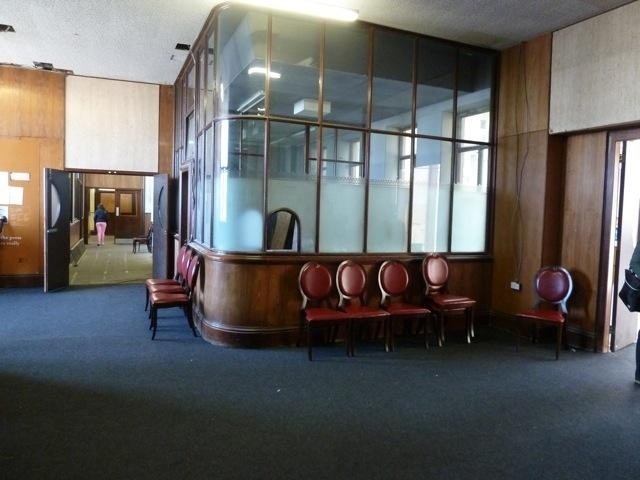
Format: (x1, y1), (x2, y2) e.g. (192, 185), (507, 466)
(237, 89), (265, 114)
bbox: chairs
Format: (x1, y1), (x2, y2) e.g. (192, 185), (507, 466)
(513, 265), (574, 360)
(145, 244), (204, 340)
(132, 223), (153, 254)
(298, 253), (477, 360)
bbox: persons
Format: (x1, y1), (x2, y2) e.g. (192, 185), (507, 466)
(629, 239), (640, 385)
(93, 203), (109, 247)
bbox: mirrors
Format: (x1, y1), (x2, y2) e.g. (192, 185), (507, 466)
(267, 208), (301, 253)
